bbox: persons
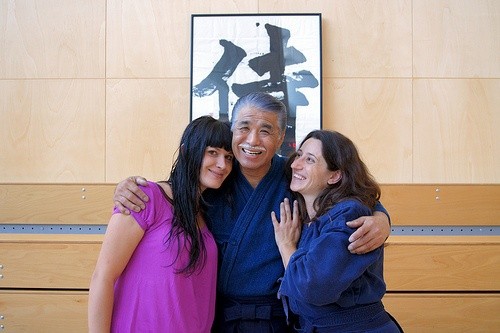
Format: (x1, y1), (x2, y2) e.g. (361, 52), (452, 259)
(112, 92), (391, 332)
(89, 116), (235, 333)
(271, 130), (402, 332)
